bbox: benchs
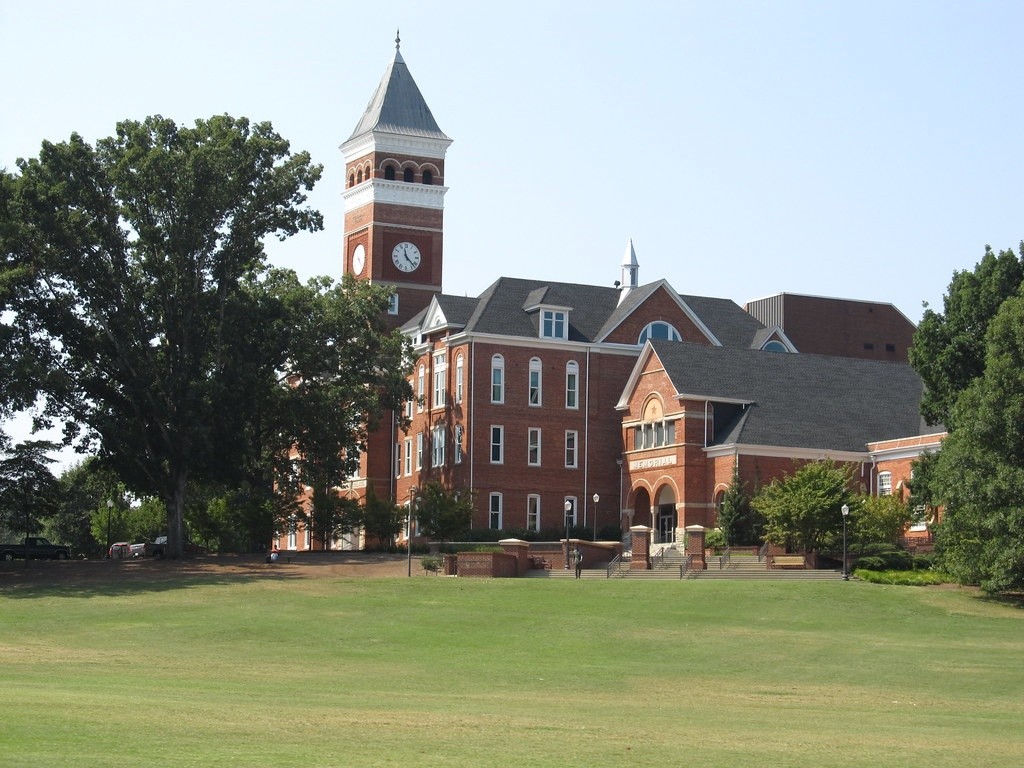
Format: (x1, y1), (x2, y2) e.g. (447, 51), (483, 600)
(266, 550), (297, 563)
(532, 554), (552, 570)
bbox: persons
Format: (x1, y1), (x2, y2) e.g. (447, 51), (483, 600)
(270, 545), (279, 563)
(572, 545), (583, 579)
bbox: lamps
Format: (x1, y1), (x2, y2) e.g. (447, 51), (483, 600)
(614, 281), (620, 288)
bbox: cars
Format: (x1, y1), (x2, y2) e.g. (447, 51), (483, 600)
(130, 544), (145, 558)
(110, 542), (130, 559)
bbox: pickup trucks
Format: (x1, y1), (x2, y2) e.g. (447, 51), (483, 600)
(0, 537), (71, 560)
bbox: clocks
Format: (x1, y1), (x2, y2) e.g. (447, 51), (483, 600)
(391, 242), (421, 273)
(351, 243), (365, 276)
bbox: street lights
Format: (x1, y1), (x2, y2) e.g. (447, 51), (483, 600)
(106, 498), (114, 558)
(565, 501), (572, 570)
(593, 493), (600, 541)
(841, 504), (849, 581)
